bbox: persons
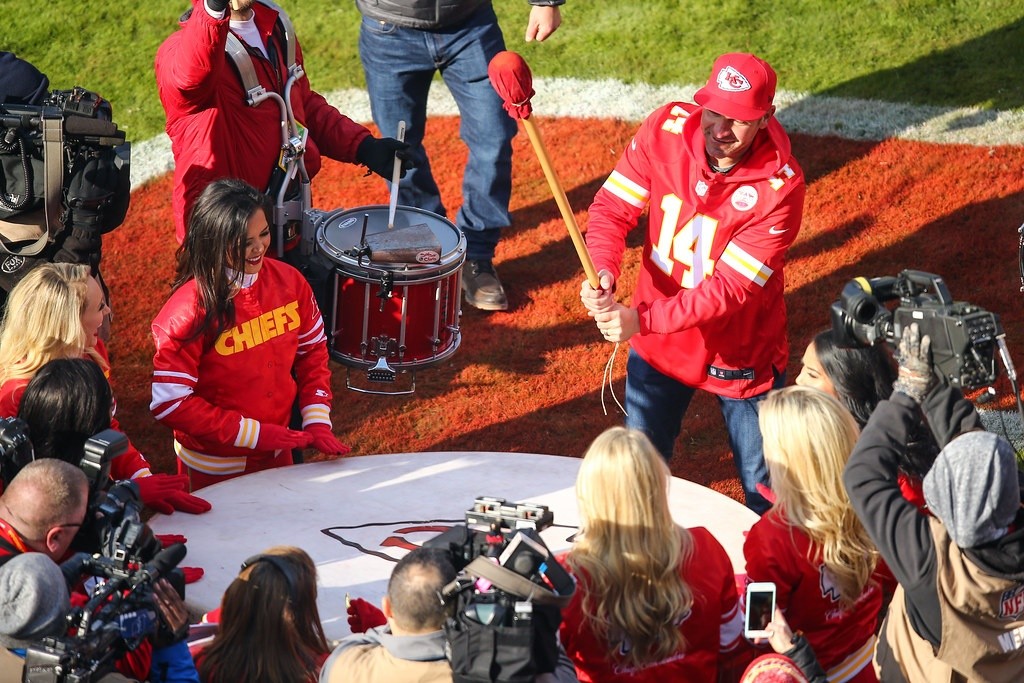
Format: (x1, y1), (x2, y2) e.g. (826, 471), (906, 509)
(321, 322), (1024, 683)
(149, 1), (424, 494)
(579, 53), (806, 516)
(0, 51), (334, 683)
(356, 0), (568, 310)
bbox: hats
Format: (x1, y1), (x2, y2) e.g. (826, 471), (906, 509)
(693, 53), (777, 121)
(0, 552), (69, 648)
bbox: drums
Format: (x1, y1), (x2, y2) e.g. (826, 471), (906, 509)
(315, 203), (468, 368)
(142, 452), (762, 683)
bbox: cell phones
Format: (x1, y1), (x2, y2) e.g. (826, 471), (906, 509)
(744, 582), (777, 639)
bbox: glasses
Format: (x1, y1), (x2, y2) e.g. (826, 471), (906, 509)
(240, 553), (298, 605)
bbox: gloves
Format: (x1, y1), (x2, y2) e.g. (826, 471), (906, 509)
(156, 490), (212, 515)
(302, 425), (352, 454)
(181, 566), (204, 584)
(893, 322), (933, 402)
(348, 598), (387, 634)
(256, 424), (316, 452)
(130, 473), (189, 508)
(158, 534), (187, 548)
(358, 134), (422, 181)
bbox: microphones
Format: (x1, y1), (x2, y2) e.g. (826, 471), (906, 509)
(65, 116), (126, 147)
(142, 542), (187, 583)
(497, 531), (549, 583)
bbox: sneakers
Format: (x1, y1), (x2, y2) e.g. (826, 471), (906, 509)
(460, 259), (509, 310)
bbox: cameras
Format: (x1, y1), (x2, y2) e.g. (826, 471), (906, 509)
(78, 428), (145, 535)
(0, 417), (36, 483)
